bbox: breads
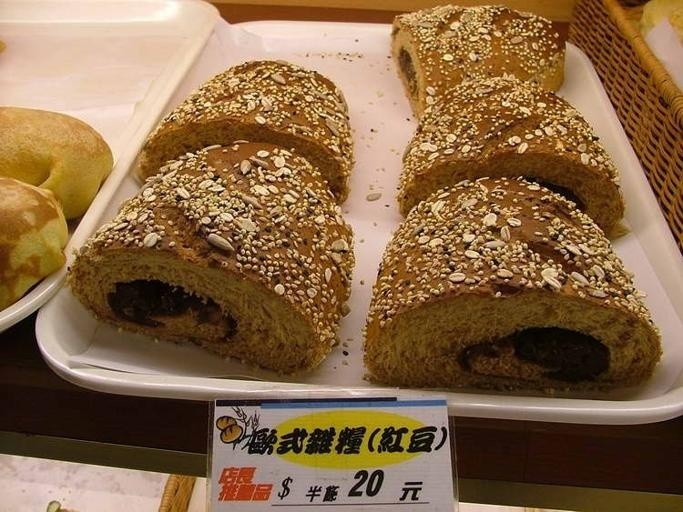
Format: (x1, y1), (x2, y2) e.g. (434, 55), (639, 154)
(397, 76), (630, 239)
(392, 4), (566, 119)
(362, 175), (662, 393)
(135, 60), (354, 204)
(72, 143), (353, 373)
(0, 176), (69, 313)
(0, 107), (114, 220)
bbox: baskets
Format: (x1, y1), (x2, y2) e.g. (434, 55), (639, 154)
(569, 0), (683, 253)
